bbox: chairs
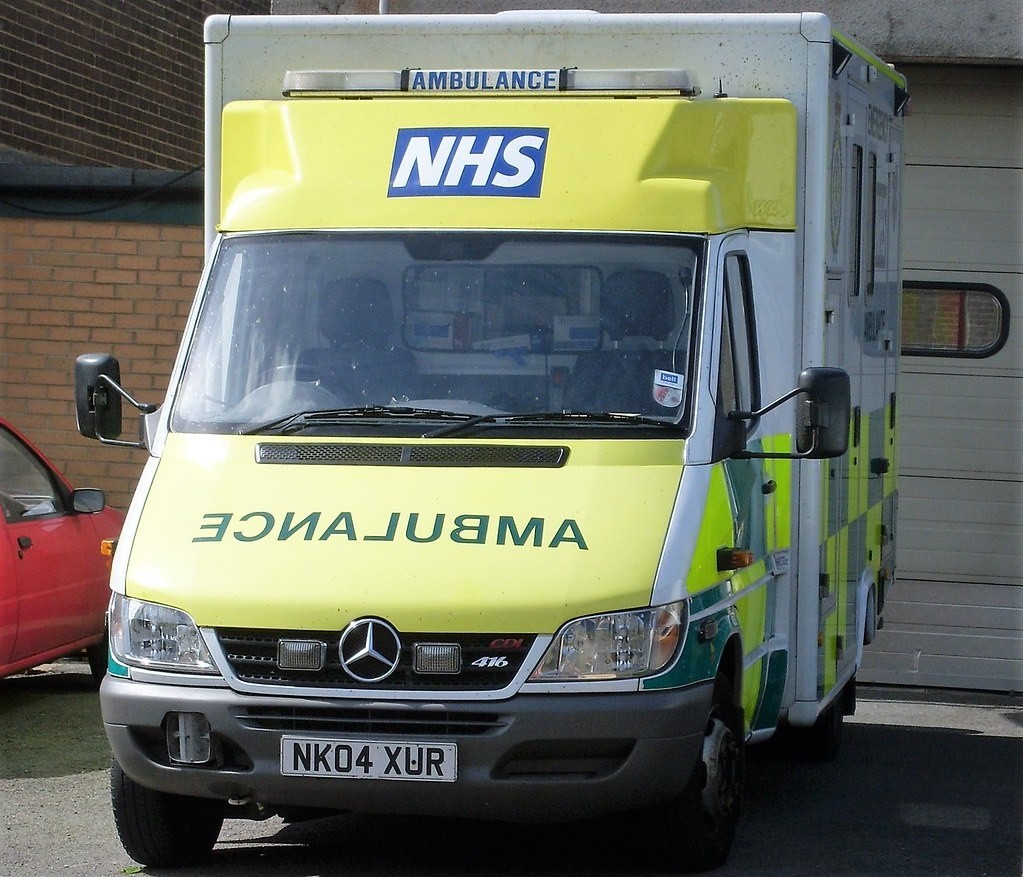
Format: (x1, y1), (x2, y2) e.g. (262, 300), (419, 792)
(295, 277), (421, 409)
(560, 269), (685, 417)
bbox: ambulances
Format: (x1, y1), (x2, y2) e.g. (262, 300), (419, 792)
(73, 8), (911, 873)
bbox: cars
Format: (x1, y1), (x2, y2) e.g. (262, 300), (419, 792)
(0, 417), (126, 679)
